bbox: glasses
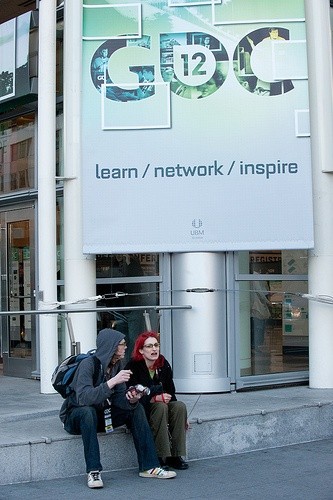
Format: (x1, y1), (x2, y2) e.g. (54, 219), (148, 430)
(119, 342), (126, 347)
(142, 343), (159, 349)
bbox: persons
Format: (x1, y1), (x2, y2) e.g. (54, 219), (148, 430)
(124, 332), (190, 469)
(59, 328), (177, 489)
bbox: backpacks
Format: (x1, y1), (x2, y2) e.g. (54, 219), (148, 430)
(51, 349), (120, 399)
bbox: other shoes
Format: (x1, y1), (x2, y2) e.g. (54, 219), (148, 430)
(158, 456), (169, 471)
(172, 456), (188, 469)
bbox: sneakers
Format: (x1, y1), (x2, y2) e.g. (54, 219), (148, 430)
(88, 471), (103, 487)
(139, 467), (176, 478)
